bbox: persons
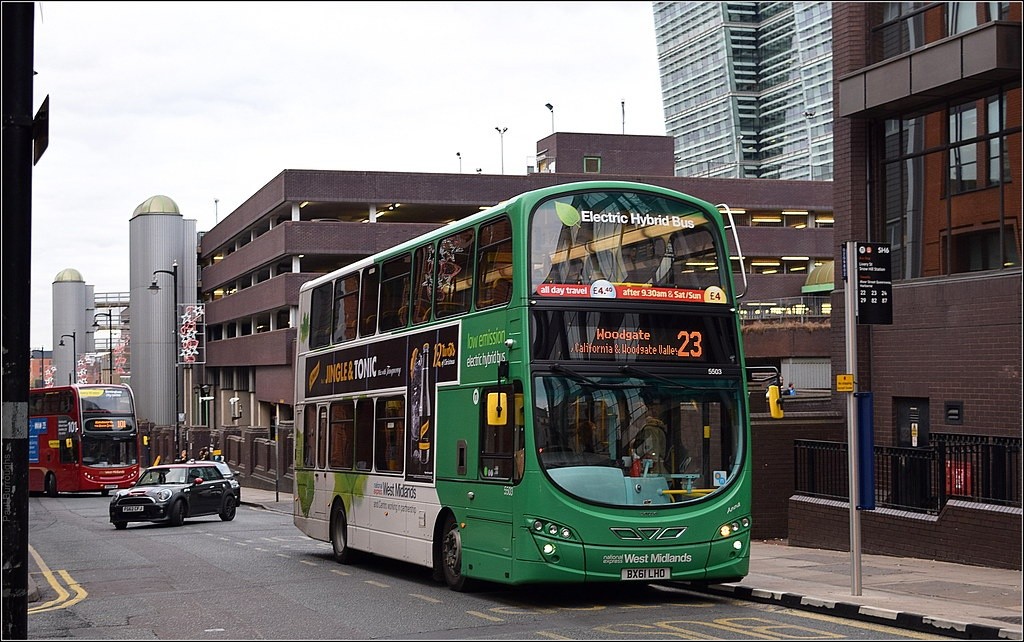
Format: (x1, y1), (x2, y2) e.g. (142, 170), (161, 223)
(621, 397), (668, 458)
(623, 245), (645, 271)
(189, 469), (200, 482)
(162, 454), (172, 464)
(198, 446), (209, 460)
(578, 421), (604, 453)
(785, 382), (795, 395)
(94, 452), (111, 464)
(181, 449), (185, 460)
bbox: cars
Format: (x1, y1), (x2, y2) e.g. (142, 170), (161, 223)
(109, 460), (241, 532)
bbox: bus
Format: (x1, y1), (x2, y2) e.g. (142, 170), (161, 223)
(29, 383), (141, 497)
(288, 180), (755, 595)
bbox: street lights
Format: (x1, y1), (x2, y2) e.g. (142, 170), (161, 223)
(91, 309), (113, 385)
(147, 258), (181, 465)
(30, 347), (44, 387)
(58, 331), (77, 383)
